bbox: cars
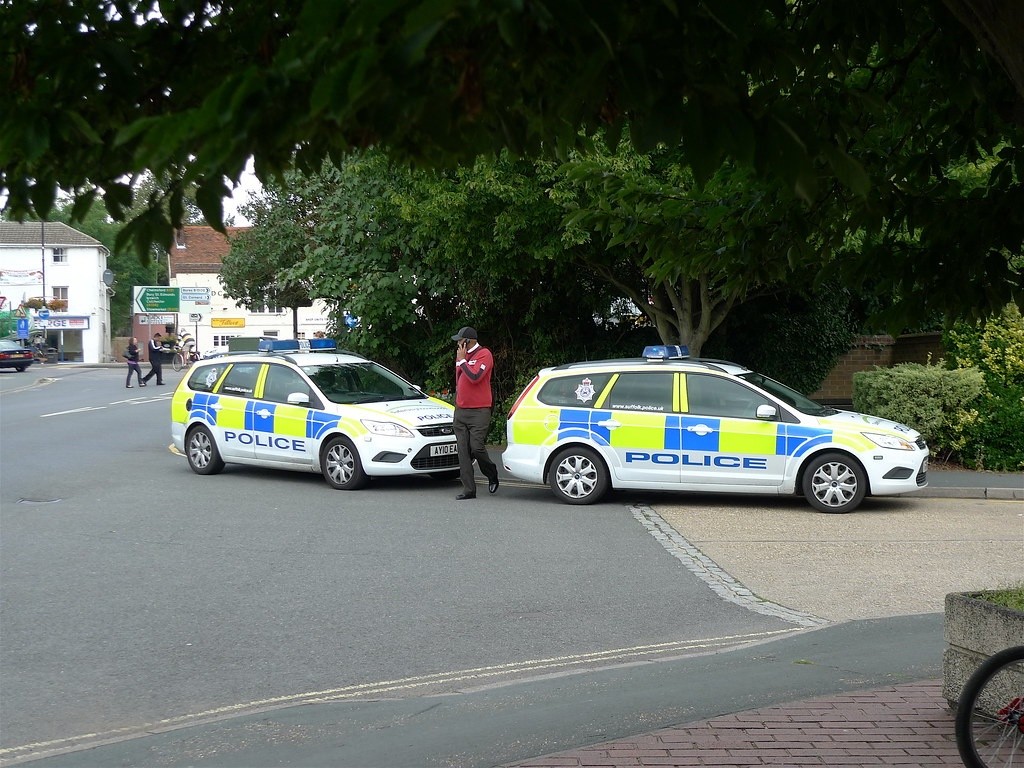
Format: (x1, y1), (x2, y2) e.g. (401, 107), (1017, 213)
(0, 339), (34, 371)
(169, 338), (477, 491)
(502, 345), (930, 514)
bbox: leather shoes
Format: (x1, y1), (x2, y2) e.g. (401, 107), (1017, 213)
(488, 475), (499, 493)
(456, 491), (476, 500)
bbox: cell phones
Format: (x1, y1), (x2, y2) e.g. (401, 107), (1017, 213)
(462, 338), (471, 349)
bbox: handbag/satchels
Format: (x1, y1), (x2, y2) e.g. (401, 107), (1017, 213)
(122, 350), (133, 359)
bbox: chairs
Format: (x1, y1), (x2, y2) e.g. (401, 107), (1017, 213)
(319, 371), (343, 395)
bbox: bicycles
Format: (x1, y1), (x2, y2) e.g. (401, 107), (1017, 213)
(172, 346), (200, 372)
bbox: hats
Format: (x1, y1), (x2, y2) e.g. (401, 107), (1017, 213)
(452, 327), (478, 341)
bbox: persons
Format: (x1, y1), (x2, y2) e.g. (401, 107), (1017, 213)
(143, 332), (166, 385)
(451, 327), (499, 500)
(126, 337), (147, 388)
(175, 328), (195, 366)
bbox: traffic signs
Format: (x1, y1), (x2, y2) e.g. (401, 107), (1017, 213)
(133, 286), (211, 323)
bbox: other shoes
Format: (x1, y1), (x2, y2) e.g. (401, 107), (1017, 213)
(139, 383), (146, 387)
(142, 378), (146, 384)
(157, 382), (165, 385)
(126, 384), (133, 388)
(184, 363), (188, 367)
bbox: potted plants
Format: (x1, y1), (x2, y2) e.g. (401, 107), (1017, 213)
(160, 349), (177, 365)
(158, 335), (177, 348)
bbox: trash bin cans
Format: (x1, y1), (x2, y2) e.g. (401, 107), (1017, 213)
(46, 348), (58, 363)
